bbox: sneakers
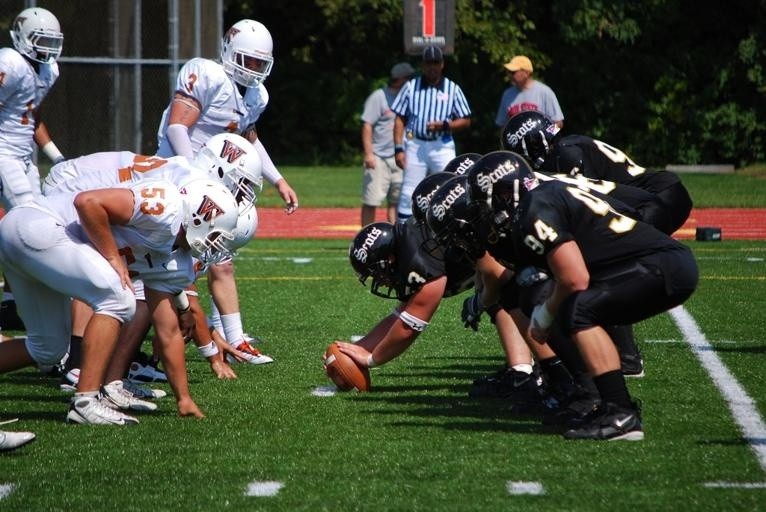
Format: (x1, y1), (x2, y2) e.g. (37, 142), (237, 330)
(122, 381), (167, 398)
(0, 430), (35, 450)
(534, 365), (545, 388)
(98, 382), (157, 412)
(469, 369), (542, 397)
(129, 362), (171, 382)
(224, 341), (273, 365)
(621, 354), (645, 377)
(65, 397), (139, 426)
(59, 371), (82, 392)
(564, 406), (645, 442)
(541, 393), (603, 425)
(508, 392), (564, 418)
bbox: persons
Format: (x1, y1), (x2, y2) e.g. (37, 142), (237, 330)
(427, 176), (645, 414)
(127, 201), (258, 382)
(495, 55), (563, 152)
(466, 150), (699, 441)
(42, 134), (265, 414)
(321, 218), (546, 401)
(0, 183), (238, 426)
(392, 46), (473, 225)
(413, 164), (669, 400)
(0, 7), (65, 208)
(159, 19), (298, 366)
(361, 65), (418, 229)
(500, 111), (692, 237)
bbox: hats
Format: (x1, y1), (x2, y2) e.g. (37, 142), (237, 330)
(389, 64), (418, 81)
(505, 56), (534, 72)
(422, 46), (443, 62)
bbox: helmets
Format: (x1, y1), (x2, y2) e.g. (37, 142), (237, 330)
(427, 172), (492, 257)
(199, 134), (263, 216)
(227, 197), (259, 249)
(412, 173), (459, 261)
(350, 222), (405, 301)
(467, 149), (540, 242)
(222, 20), (273, 87)
(502, 112), (564, 170)
(179, 173), (238, 258)
(442, 153), (485, 176)
(10, 7), (63, 64)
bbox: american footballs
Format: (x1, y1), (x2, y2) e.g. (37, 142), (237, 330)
(326, 342), (371, 393)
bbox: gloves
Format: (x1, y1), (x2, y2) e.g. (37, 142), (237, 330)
(461, 294), (485, 331)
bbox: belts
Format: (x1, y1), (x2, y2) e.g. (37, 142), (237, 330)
(415, 135), (440, 141)
